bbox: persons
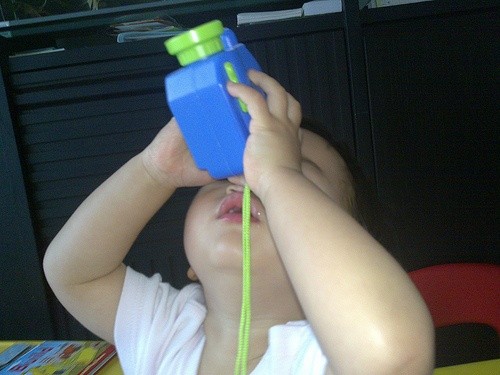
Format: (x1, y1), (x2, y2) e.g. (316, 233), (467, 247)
(42, 69), (437, 375)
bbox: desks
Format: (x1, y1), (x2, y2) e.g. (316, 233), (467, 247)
(0, 341), (123, 375)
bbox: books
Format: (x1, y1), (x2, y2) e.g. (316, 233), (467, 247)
(237, 8), (303, 25)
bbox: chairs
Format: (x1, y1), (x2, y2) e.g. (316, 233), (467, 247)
(408, 261), (500, 375)
